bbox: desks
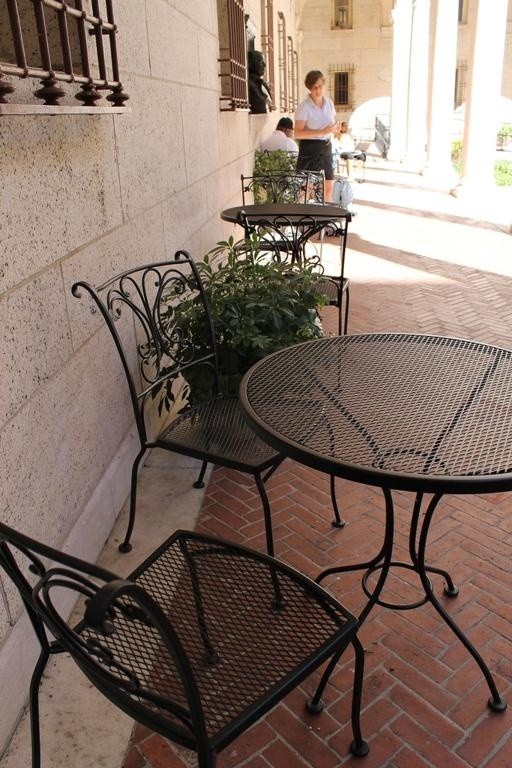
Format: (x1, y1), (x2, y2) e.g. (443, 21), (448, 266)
(239, 332), (512, 716)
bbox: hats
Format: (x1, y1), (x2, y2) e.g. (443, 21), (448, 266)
(277, 116), (293, 130)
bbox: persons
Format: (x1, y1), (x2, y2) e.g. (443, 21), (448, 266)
(335, 121), (367, 182)
(248, 50), (276, 113)
(262, 116), (317, 183)
(294, 71), (337, 204)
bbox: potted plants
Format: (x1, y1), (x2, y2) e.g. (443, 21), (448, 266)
(153, 226), (329, 404)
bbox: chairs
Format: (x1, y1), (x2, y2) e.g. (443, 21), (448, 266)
(221, 150), (356, 335)
(0, 523), (370, 767)
(71, 249), (346, 610)
